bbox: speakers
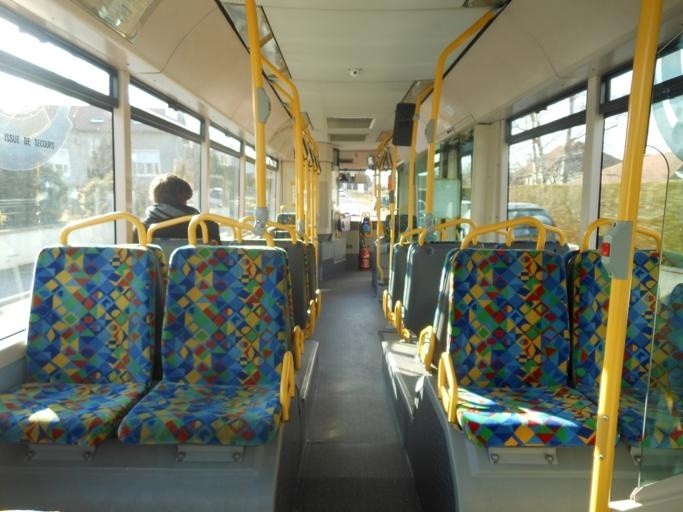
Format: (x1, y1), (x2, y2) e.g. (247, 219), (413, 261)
(392, 102), (416, 146)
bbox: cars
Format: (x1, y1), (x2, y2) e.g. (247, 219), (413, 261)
(416, 197), (559, 244)
(370, 194), (391, 216)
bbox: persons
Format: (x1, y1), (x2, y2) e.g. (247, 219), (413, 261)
(132, 175), (218, 243)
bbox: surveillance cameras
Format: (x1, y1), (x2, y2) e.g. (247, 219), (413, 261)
(349, 69), (360, 79)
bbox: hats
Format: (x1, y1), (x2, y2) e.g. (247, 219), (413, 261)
(154, 175), (192, 203)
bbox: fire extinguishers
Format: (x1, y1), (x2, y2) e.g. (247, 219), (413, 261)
(360, 244), (370, 271)
